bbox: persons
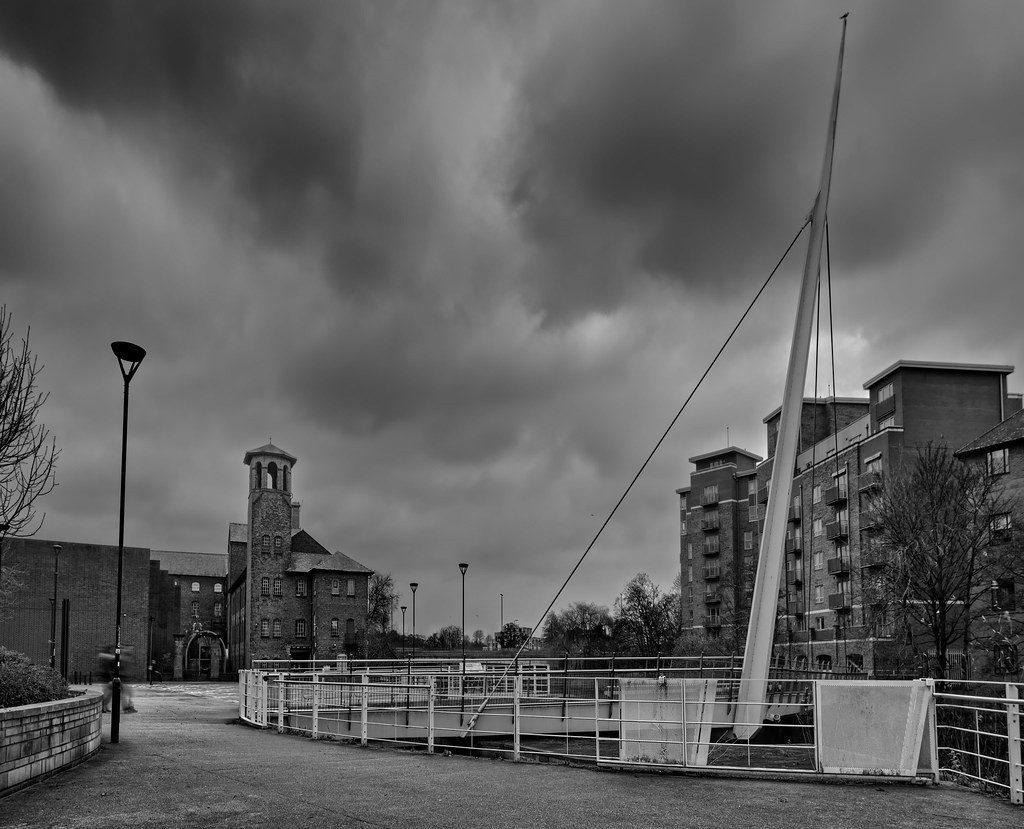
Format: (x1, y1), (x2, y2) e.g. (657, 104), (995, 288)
(149, 660), (162, 685)
(99, 646), (138, 713)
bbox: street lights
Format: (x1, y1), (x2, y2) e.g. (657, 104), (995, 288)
(400, 606), (408, 659)
(410, 582), (419, 658)
(500, 593), (504, 631)
(514, 619), (518, 625)
(50, 542), (62, 671)
(110, 342), (146, 744)
(459, 563), (469, 672)
